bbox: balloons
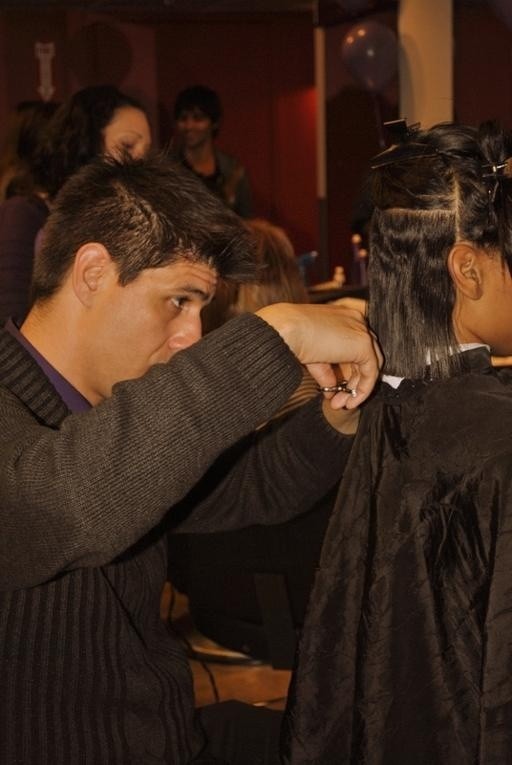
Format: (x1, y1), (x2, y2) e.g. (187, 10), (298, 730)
(342, 20), (398, 93)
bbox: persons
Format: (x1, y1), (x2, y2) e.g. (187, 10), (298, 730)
(1, 86), (308, 331)
(0, 152), (382, 764)
(281, 116), (511, 764)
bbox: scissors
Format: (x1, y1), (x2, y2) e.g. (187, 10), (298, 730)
(313, 380), (352, 394)
